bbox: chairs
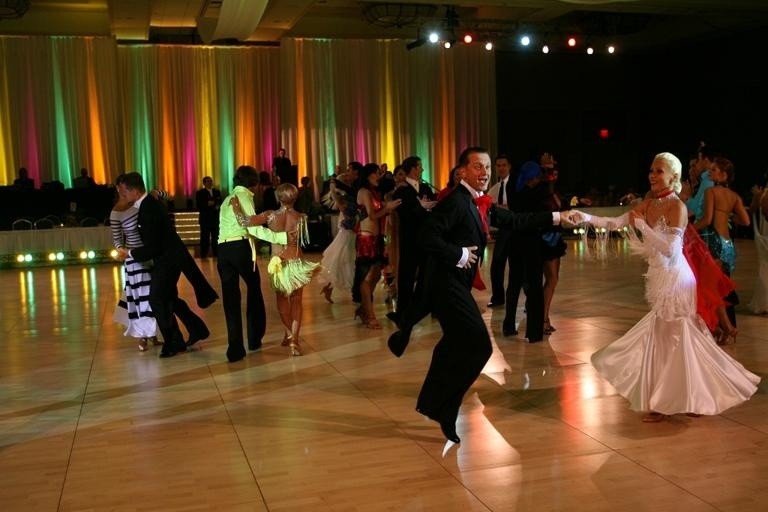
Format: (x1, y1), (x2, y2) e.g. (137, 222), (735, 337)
(35, 217), (54, 230)
(12, 218), (33, 231)
(80, 216), (100, 228)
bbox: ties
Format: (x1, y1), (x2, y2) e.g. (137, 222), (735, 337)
(415, 183), (419, 192)
(498, 181), (503, 205)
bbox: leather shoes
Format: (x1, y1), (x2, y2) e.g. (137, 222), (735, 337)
(504, 331), (519, 336)
(186, 332), (210, 346)
(487, 303), (498, 307)
(439, 419), (460, 443)
(159, 347), (186, 358)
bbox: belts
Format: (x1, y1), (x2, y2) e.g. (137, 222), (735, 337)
(218, 236), (256, 273)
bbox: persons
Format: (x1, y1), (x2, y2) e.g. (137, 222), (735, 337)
(332, 164), (341, 177)
(227, 182), (319, 356)
(215, 164), (297, 362)
(536, 151), (563, 336)
(196, 176), (223, 261)
(436, 165), (495, 290)
(109, 174), (170, 351)
(75, 168), (95, 186)
(384, 164), (407, 305)
(273, 148), (292, 168)
(680, 146), (737, 329)
(119, 171), (218, 359)
(262, 174), (282, 256)
(485, 153), (523, 308)
(330, 159), (364, 303)
(317, 168), (357, 305)
(14, 167), (33, 188)
(691, 157), (750, 348)
(354, 162), (403, 331)
(502, 159), (552, 343)
(391, 156), (440, 319)
(389, 146), (493, 444)
(572, 152), (762, 421)
(294, 176), (314, 214)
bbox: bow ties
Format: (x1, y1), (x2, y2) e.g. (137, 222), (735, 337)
(475, 195), (493, 240)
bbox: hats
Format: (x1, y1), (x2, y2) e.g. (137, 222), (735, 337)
(515, 162), (541, 192)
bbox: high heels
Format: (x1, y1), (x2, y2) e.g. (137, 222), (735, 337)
(544, 320), (556, 331)
(718, 328), (738, 345)
(281, 334), (292, 346)
(385, 293), (396, 303)
(365, 313), (383, 329)
(138, 339), (147, 351)
(686, 412), (704, 417)
(319, 286), (334, 303)
(642, 412), (679, 421)
(149, 338), (160, 346)
(354, 310), (365, 323)
(288, 341), (302, 357)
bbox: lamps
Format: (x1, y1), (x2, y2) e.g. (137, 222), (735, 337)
(180, 237), (200, 241)
(174, 211), (200, 215)
(177, 230), (201, 234)
(406, 27), (425, 51)
(439, 29), (459, 49)
(175, 224), (201, 228)
(572, 226), (629, 236)
(175, 218), (200, 221)
(464, 34), (615, 54)
(17, 250), (119, 263)
